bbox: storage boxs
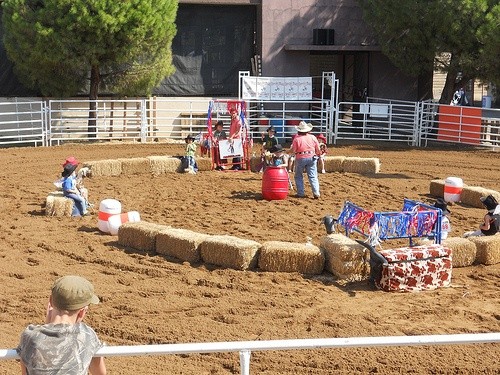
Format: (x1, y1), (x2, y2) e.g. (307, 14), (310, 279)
(377, 244), (453, 292)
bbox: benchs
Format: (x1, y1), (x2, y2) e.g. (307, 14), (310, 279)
(258, 241), (324, 274)
(429, 179), (500, 209)
(82, 155), (213, 178)
(323, 233), (371, 279)
(437, 237), (477, 267)
(118, 221), (172, 250)
(251, 152), (379, 174)
(201, 234), (261, 271)
(468, 232), (500, 265)
(156, 227), (205, 263)
(46, 191), (73, 218)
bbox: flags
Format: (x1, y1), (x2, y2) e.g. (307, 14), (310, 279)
(239, 100), (248, 145)
(207, 101), (214, 138)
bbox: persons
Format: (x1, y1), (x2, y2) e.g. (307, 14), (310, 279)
(16, 275), (106, 375)
(286, 120), (321, 199)
(61, 155), (94, 216)
(261, 126), (288, 167)
(463, 194), (500, 238)
(420, 198), (451, 240)
(288, 133), (327, 173)
(227, 108), (242, 170)
(185, 135), (196, 168)
(214, 122), (228, 164)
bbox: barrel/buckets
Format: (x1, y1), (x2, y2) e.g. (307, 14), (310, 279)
(261, 166), (289, 201)
(444, 176), (463, 202)
(108, 211), (140, 235)
(97, 199), (121, 233)
(258, 115), (300, 142)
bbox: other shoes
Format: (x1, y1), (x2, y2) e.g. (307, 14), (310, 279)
(314, 195), (319, 198)
(321, 169), (325, 173)
(295, 194), (305, 198)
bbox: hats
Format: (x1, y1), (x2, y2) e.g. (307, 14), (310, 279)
(215, 120), (225, 127)
(62, 163), (78, 178)
(431, 198), (451, 215)
(267, 127), (277, 134)
(316, 134), (326, 142)
(63, 159), (76, 167)
(52, 276), (99, 311)
(479, 194), (498, 209)
(295, 121), (313, 133)
(185, 135), (195, 140)
